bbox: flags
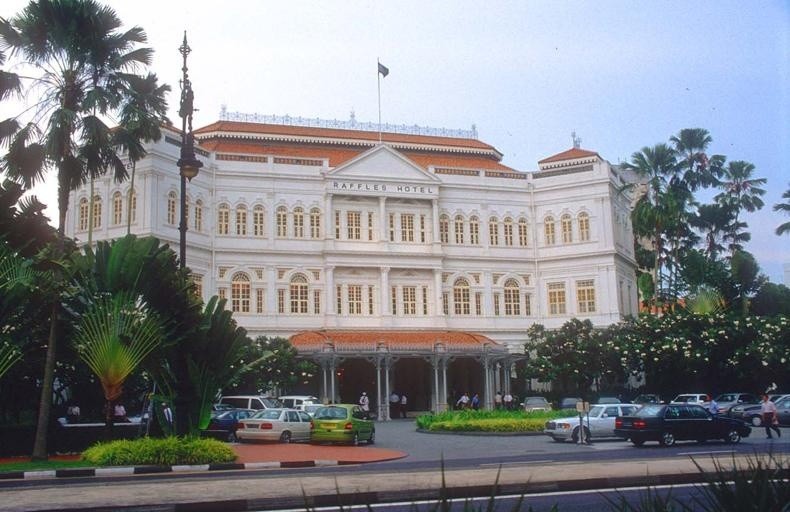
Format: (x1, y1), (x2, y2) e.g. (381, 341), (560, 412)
(378, 62), (389, 77)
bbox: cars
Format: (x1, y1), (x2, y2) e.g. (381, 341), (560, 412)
(199, 395), (376, 445)
(518, 393), (790, 447)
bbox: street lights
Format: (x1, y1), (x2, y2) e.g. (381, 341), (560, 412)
(173, 29), (206, 441)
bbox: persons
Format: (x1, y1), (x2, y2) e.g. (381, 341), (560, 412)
(761, 394), (781, 439)
(67, 401), (81, 424)
(114, 400), (127, 422)
(359, 391), (513, 419)
(706, 392), (719, 414)
(161, 402), (173, 424)
